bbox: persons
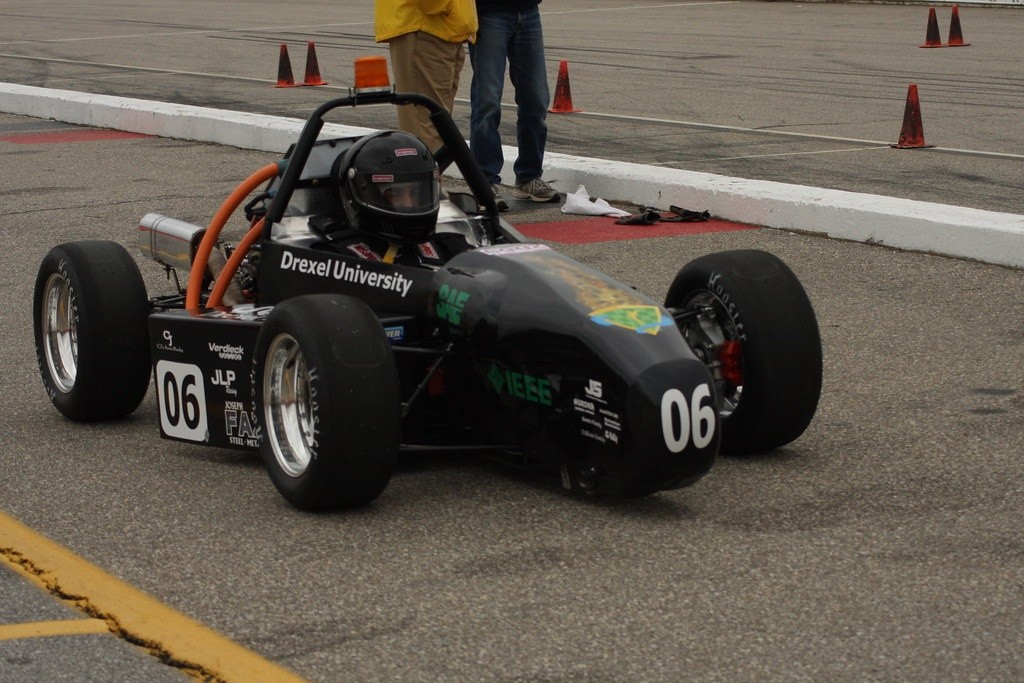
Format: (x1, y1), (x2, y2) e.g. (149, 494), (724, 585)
(375, 0), (479, 157)
(468, 0), (561, 214)
(316, 132), (464, 273)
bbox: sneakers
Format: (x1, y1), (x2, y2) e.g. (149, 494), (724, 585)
(479, 183), (508, 211)
(512, 176), (561, 204)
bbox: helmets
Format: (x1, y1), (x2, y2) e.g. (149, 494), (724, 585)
(340, 129), (440, 247)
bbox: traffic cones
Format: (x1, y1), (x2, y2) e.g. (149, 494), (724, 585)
(273, 44), (303, 88)
(917, 6), (947, 48)
(946, 3), (972, 47)
(548, 60), (584, 113)
(887, 83), (937, 149)
(302, 41), (329, 86)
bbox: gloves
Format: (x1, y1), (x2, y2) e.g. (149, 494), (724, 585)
(659, 206), (710, 223)
(615, 205), (660, 225)
